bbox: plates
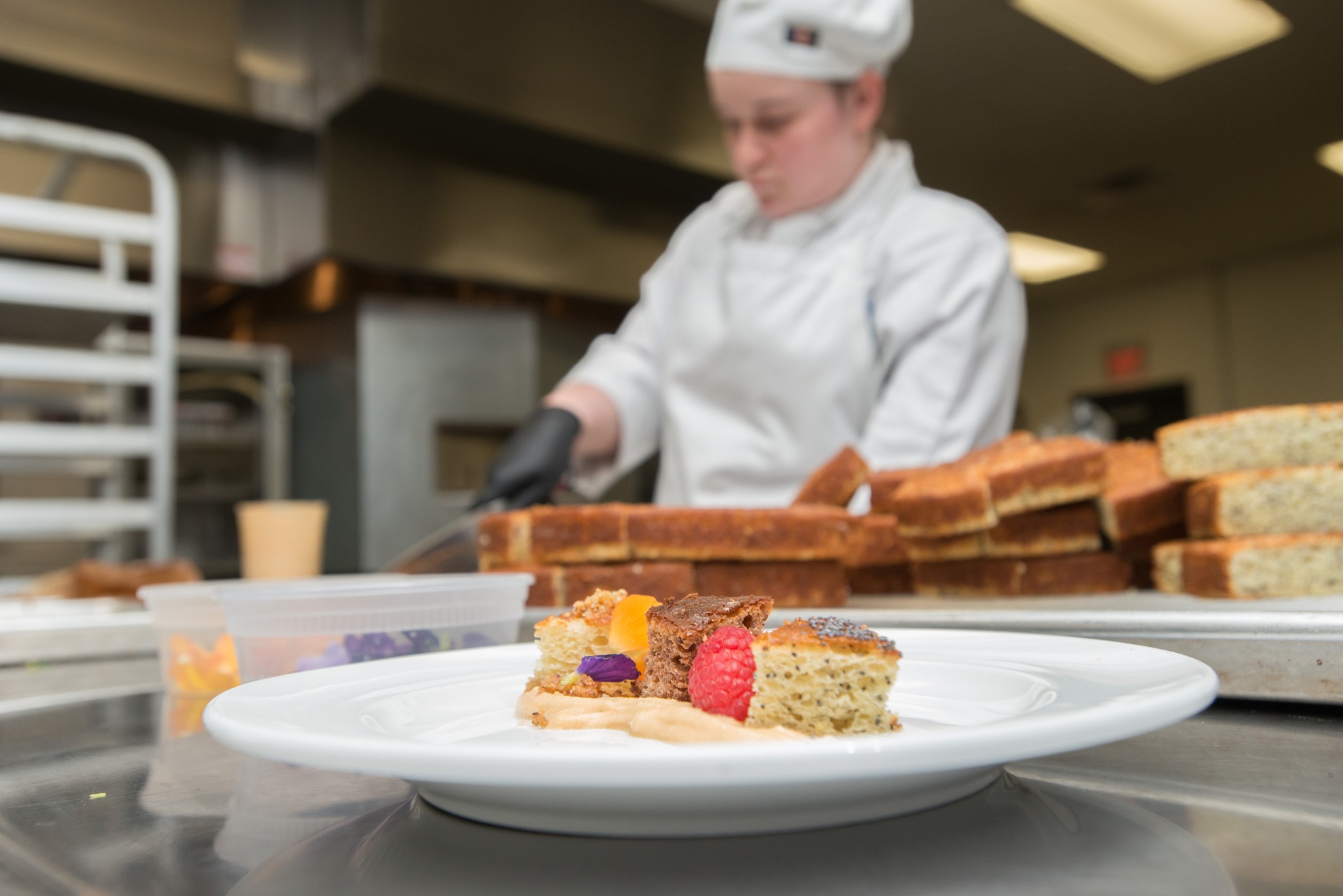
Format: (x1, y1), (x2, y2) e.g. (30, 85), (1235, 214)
(201, 629), (1221, 837)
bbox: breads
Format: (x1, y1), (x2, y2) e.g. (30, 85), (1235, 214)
(26, 554), (199, 599)
(478, 399), (1343, 610)
(515, 587), (901, 746)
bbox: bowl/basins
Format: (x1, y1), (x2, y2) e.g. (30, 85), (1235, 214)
(137, 572), (536, 690)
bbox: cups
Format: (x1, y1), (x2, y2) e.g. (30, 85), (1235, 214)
(235, 499), (329, 579)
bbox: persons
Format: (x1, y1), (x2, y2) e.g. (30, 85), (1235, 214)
(545, 0), (1030, 514)
